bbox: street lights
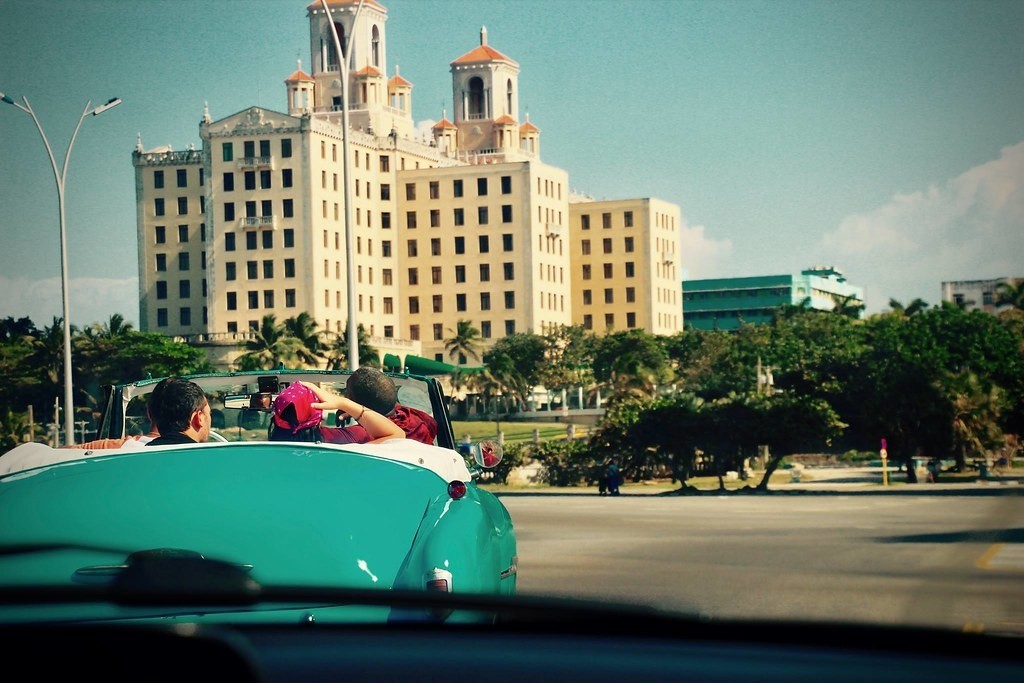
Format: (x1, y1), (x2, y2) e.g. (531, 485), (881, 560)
(0, 93), (122, 445)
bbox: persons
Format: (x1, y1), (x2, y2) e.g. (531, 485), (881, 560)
(267, 380), (406, 444)
(120, 400), (161, 449)
(598, 458), (620, 496)
(335, 367), (437, 446)
(250, 394), (272, 408)
(143, 376), (212, 446)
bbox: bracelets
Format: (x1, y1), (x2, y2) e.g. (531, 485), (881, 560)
(354, 405), (365, 421)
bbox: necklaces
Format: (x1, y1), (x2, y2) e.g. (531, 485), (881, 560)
(150, 432), (160, 435)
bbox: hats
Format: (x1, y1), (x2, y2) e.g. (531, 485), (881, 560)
(274, 381), (323, 433)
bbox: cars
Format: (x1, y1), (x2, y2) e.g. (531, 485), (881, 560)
(0, 362), (518, 629)
